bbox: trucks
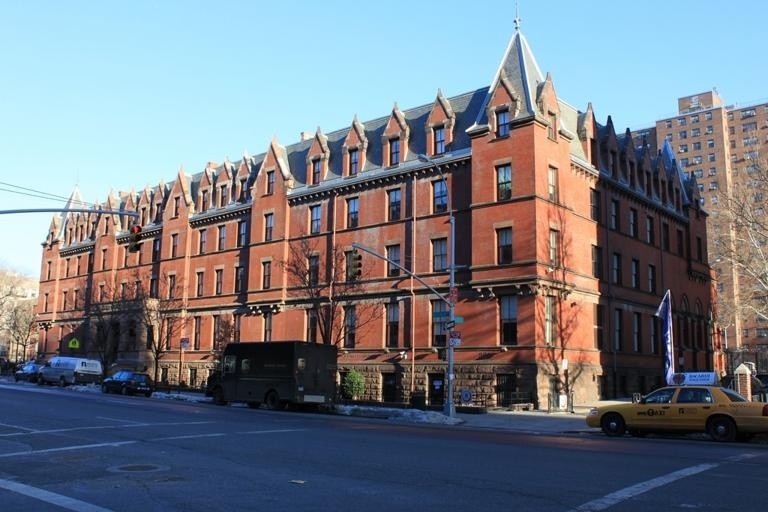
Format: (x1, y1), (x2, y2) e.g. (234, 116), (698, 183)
(204, 339), (340, 413)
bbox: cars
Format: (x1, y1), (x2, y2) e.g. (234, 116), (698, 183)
(101, 369), (152, 399)
(13, 364), (45, 384)
(584, 383), (768, 443)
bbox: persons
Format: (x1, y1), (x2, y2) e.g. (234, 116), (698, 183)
(720, 371), (731, 389)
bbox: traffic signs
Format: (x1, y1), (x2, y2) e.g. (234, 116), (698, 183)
(453, 317), (463, 323)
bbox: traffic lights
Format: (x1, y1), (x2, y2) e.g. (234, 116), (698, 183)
(350, 254), (362, 278)
(128, 224), (144, 253)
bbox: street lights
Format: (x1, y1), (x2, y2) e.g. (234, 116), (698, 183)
(39, 322), (51, 352)
(417, 153), (462, 421)
(245, 303), (283, 342)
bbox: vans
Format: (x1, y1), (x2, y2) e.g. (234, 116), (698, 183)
(37, 358), (102, 389)
